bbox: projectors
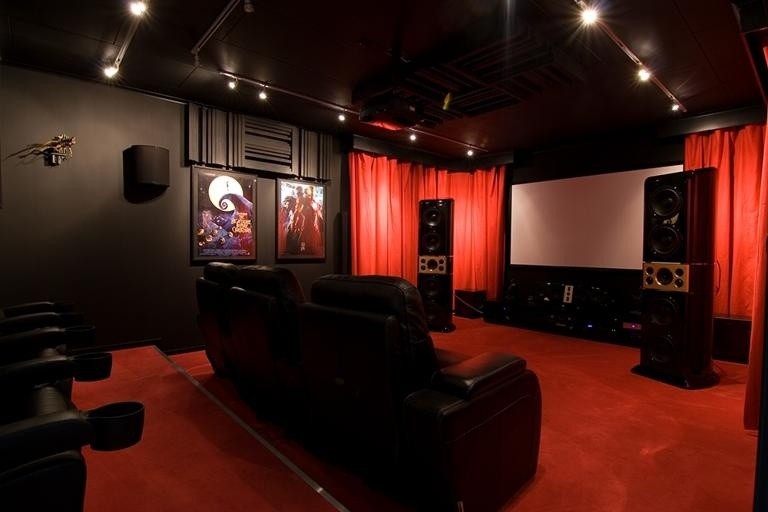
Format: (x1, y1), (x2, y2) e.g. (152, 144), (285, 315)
(358, 95), (426, 131)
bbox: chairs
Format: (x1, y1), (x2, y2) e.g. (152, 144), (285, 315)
(0, 295), (89, 511)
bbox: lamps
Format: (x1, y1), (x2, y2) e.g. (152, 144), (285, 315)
(190, 1), (254, 66)
(576, 0), (689, 115)
(101, 1), (149, 86)
(219, 72), (489, 154)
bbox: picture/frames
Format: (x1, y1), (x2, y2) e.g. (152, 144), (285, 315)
(189, 164), (258, 265)
(275, 176), (328, 264)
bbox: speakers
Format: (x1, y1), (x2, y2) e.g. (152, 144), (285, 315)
(630, 168), (720, 390)
(418, 198), (455, 332)
(130, 144), (169, 185)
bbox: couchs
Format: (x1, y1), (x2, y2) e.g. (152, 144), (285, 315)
(195, 261), (542, 511)
